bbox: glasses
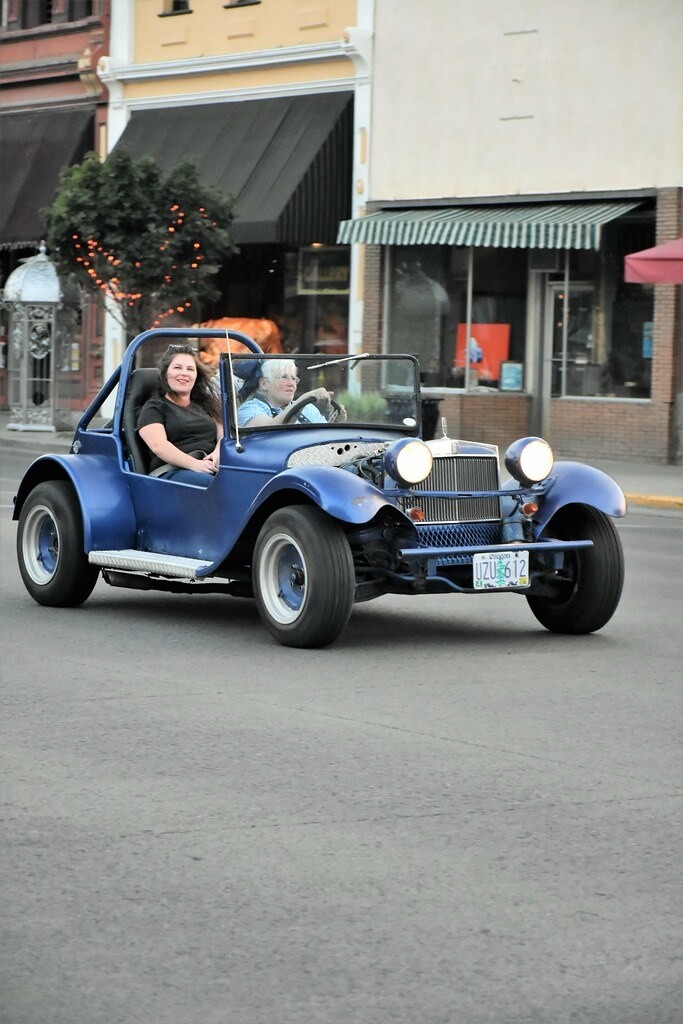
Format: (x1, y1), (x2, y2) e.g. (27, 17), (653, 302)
(264, 374), (300, 385)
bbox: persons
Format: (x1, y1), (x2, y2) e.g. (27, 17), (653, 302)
(137, 344), (231, 488)
(236, 359), (347, 428)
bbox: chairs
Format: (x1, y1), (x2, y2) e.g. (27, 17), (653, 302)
(124, 368), (159, 473)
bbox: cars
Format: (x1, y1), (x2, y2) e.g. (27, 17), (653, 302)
(11, 328), (632, 649)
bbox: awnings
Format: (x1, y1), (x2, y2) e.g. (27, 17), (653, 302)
(336, 200), (644, 252)
(625, 238), (683, 285)
(107, 91), (353, 247)
(0, 110), (96, 249)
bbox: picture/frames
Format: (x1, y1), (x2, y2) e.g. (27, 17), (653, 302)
(498, 360), (525, 393)
